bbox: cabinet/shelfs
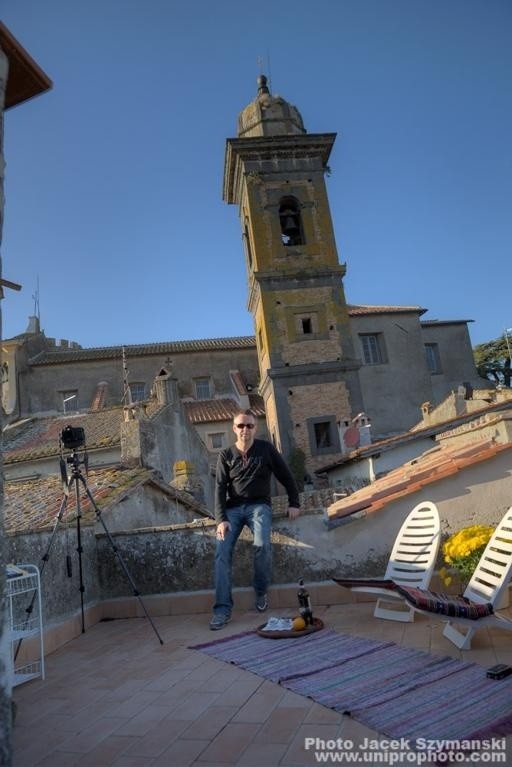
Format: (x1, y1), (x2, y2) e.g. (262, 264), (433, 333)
(0, 563), (44, 689)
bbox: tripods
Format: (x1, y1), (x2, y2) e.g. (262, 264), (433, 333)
(14, 449), (164, 662)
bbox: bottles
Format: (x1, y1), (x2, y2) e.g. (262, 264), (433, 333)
(297, 580), (315, 626)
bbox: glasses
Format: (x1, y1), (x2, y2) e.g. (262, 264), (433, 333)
(242, 453), (248, 469)
(235, 424), (255, 429)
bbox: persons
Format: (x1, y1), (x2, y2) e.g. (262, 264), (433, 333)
(207, 413), (301, 632)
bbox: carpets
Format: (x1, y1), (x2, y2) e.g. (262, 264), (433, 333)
(187, 621), (512, 767)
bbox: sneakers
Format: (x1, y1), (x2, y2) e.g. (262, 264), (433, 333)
(254, 593), (269, 613)
(209, 613), (232, 630)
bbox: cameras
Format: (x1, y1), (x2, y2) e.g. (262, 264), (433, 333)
(58, 424), (85, 450)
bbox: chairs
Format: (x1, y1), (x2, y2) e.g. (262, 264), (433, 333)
(329, 501), (512, 651)
